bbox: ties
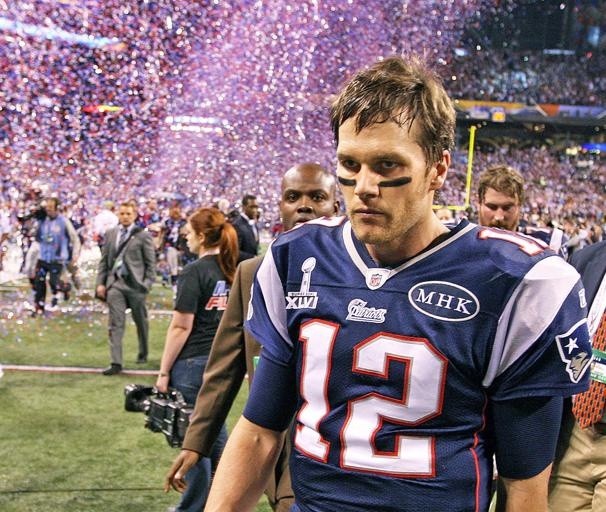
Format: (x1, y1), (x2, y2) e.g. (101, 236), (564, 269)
(115, 228), (128, 279)
(572, 305), (606, 430)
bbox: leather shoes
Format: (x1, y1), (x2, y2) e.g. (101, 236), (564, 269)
(103, 358), (147, 375)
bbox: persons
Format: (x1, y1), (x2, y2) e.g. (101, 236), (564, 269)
(1, 56), (605, 512)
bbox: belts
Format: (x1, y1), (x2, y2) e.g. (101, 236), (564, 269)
(590, 421), (605, 435)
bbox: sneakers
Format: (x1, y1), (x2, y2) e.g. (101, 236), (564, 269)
(30, 278), (81, 318)
